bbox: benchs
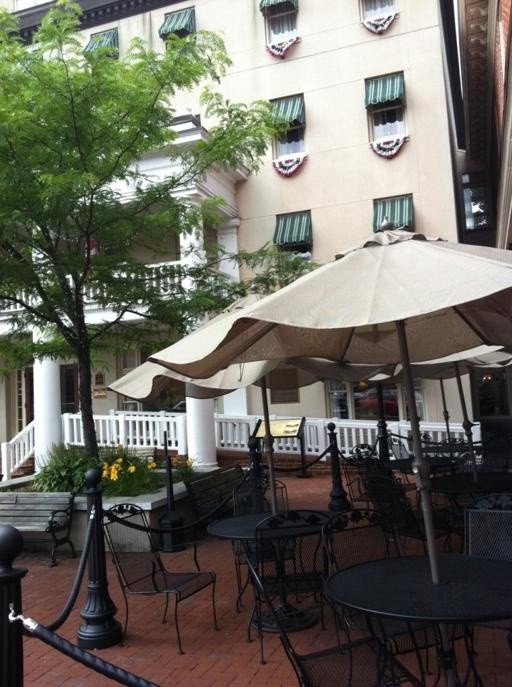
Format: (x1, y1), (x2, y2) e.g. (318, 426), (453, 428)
(1, 491), (79, 567)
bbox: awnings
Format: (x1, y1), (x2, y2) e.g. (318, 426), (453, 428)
(273, 209), (312, 245)
(373, 193), (415, 233)
